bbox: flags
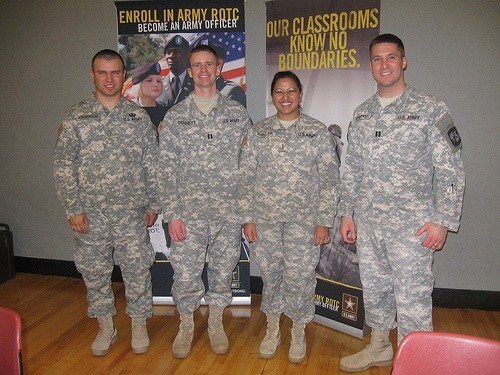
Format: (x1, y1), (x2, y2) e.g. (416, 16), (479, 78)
(120, 32), (246, 101)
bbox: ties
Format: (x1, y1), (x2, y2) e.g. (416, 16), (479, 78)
(172, 76), (180, 101)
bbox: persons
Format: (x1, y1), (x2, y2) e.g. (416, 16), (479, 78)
(338, 33), (465, 373)
(132, 35), (247, 108)
(157, 45), (253, 358)
(238, 71), (341, 363)
(53, 50), (164, 356)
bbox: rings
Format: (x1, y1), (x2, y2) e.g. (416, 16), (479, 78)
(433, 244), (438, 247)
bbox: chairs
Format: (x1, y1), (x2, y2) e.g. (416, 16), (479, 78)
(0, 306), (23, 375)
(391, 331), (500, 375)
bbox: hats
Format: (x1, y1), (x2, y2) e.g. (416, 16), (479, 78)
(131, 62), (161, 85)
(164, 35), (190, 57)
(194, 38), (227, 59)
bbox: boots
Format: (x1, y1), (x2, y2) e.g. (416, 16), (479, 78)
(90, 316), (119, 356)
(130, 318), (150, 354)
(207, 306), (229, 355)
(288, 321), (307, 363)
(171, 313), (195, 359)
(338, 328), (394, 373)
(259, 316), (282, 359)
